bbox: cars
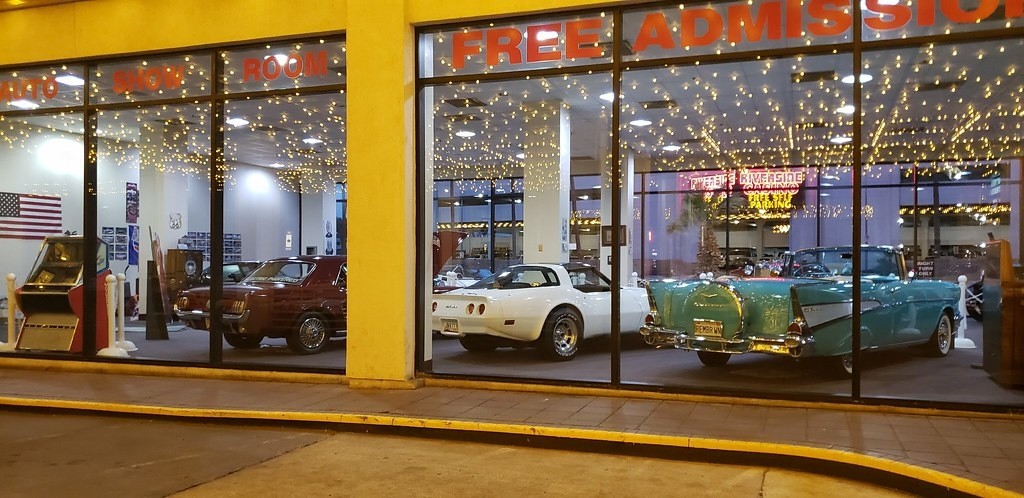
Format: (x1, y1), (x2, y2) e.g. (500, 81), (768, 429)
(728, 250), (752, 268)
(183, 261), (286, 287)
(439, 262), (493, 289)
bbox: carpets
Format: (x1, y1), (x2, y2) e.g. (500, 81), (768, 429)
(116, 326), (185, 331)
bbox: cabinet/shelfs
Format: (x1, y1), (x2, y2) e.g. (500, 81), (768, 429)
(167, 250), (203, 321)
(983, 240), (1024, 385)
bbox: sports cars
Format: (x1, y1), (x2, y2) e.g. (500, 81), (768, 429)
(174, 256), (348, 356)
(638, 247), (964, 376)
(432, 262), (649, 363)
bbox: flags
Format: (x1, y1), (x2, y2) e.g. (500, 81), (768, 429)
(0, 192), (64, 240)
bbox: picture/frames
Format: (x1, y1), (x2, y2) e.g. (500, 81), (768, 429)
(602, 226), (626, 246)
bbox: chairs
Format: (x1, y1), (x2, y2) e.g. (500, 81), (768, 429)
(796, 264), (829, 276)
